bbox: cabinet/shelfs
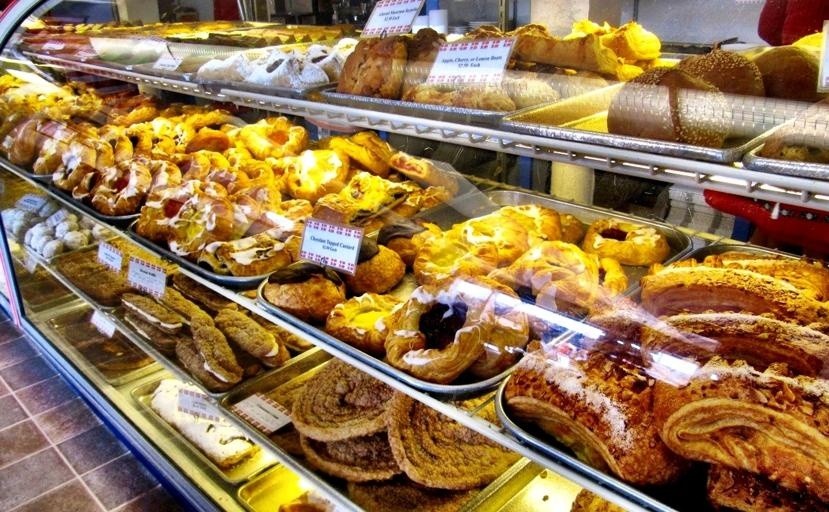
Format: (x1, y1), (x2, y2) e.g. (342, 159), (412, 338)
(1, 0), (829, 512)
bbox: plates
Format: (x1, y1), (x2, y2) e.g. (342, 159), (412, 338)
(413, 9), (514, 36)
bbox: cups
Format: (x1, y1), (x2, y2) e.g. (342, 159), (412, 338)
(330, 1), (374, 25)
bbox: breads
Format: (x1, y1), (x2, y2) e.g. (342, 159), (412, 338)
(0, 18), (829, 165)
(1, 75), (829, 510)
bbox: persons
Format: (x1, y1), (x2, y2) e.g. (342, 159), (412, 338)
(756, 1), (829, 52)
